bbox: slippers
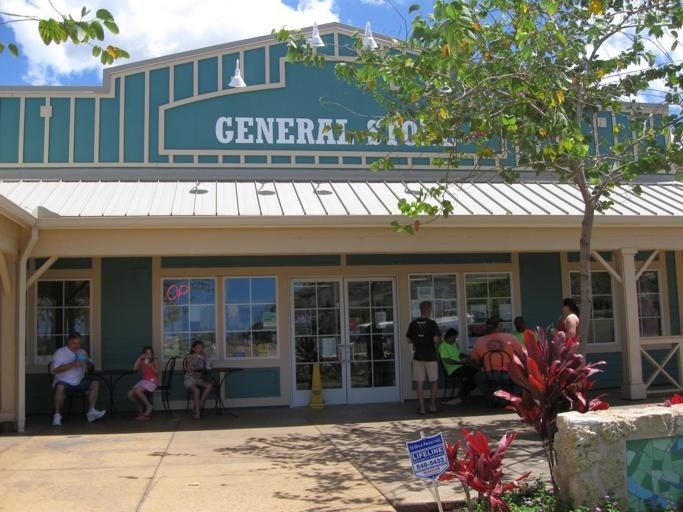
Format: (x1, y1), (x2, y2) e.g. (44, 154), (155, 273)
(135, 413), (150, 421)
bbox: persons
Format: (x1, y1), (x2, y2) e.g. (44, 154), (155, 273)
(511, 317), (540, 347)
(182, 339), (213, 421)
(126, 345), (160, 422)
(405, 300), (442, 416)
(468, 317), (528, 411)
(49, 331), (106, 427)
(552, 298), (581, 340)
(438, 327), (477, 404)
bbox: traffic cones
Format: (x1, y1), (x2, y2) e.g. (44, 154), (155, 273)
(307, 362), (327, 408)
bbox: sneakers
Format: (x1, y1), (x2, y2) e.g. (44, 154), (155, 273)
(86, 410), (106, 422)
(192, 407), (201, 419)
(51, 415), (62, 426)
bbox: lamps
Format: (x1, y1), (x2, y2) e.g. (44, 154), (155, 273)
(362, 22), (378, 49)
(309, 22), (325, 47)
(226, 58), (246, 88)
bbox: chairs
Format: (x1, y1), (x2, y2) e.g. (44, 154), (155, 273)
(140, 355), (176, 420)
(47, 360), (89, 427)
(182, 354), (217, 416)
(437, 350), (516, 413)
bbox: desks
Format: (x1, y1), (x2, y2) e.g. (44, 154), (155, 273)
(88, 367), (137, 422)
(201, 366), (242, 420)
